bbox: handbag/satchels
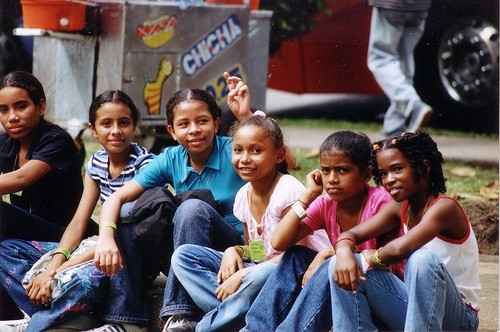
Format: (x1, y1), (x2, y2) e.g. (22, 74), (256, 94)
(124, 185), (226, 289)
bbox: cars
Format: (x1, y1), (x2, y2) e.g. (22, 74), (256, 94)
(200, 0), (499, 133)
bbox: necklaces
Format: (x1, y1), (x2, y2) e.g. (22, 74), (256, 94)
(250, 171), (276, 236)
(406, 194), (432, 228)
(337, 190), (365, 227)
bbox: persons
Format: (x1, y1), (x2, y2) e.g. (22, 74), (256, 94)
(80, 73), (296, 332)
(241, 130), (405, 332)
(328, 130), (481, 332)
(170, 114), (335, 332)
(365, 0), (433, 137)
(0, 90), (158, 332)
(0, 71), (101, 320)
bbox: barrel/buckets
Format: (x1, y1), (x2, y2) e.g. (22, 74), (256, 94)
(21, 0), (87, 32)
(206, 0), (259, 11)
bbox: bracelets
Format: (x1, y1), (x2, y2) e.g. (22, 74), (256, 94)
(52, 249), (71, 261)
(298, 200), (309, 207)
(291, 202), (307, 220)
(236, 245), (248, 262)
(333, 238), (356, 253)
(99, 222), (117, 236)
(373, 247), (392, 268)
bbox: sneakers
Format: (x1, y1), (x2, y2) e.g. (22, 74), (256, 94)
(161, 313), (198, 332)
(84, 319), (149, 332)
(0, 314), (32, 332)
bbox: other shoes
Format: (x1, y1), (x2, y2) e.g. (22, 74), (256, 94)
(377, 126), (406, 138)
(405, 102), (434, 133)
